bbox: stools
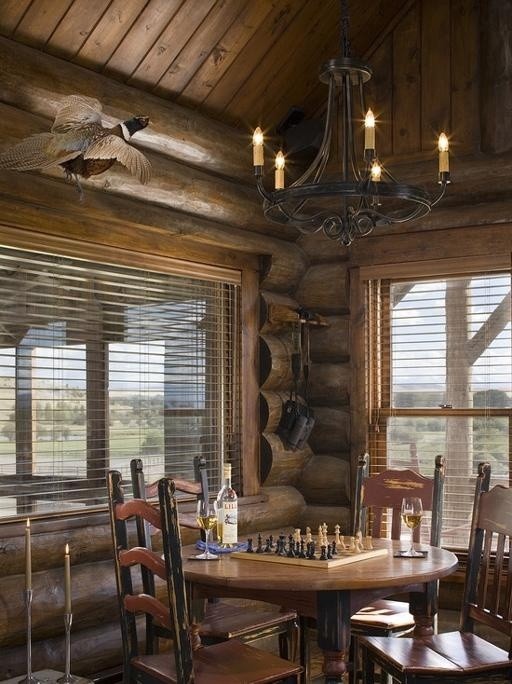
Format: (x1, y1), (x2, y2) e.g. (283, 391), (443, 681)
(0, 666), (93, 684)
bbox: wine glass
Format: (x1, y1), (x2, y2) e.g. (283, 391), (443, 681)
(196, 498), (219, 560)
(400, 495), (424, 557)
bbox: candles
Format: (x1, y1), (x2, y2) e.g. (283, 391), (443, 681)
(64, 543), (72, 616)
(24, 517), (33, 590)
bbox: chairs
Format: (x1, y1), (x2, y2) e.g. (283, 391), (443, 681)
(131, 455), (301, 664)
(349, 461), (511, 683)
(107, 470), (306, 682)
(319, 454), (446, 660)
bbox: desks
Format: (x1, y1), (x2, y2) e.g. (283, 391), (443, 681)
(158, 531), (459, 681)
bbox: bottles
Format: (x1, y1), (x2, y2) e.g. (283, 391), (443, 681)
(215, 462), (240, 550)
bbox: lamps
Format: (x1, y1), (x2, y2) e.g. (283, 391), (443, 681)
(251, 1), (450, 249)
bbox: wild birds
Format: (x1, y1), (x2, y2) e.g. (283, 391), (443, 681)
(0, 93), (154, 201)
